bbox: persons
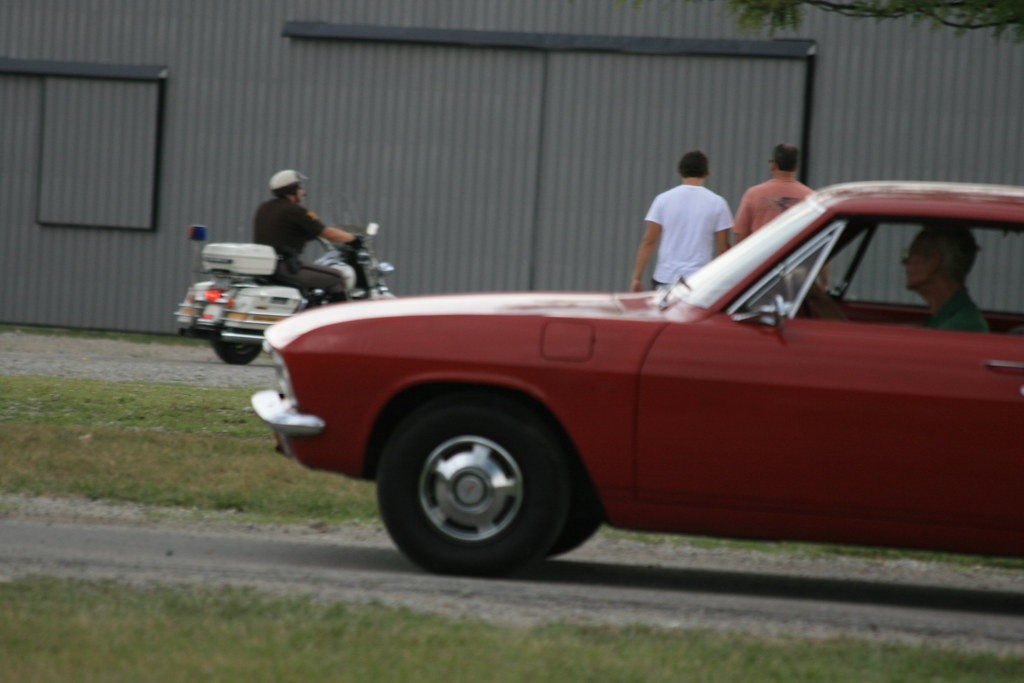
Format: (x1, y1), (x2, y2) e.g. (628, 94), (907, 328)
(251, 168), (362, 304)
(628, 149), (734, 293)
(729, 143), (831, 294)
(795, 225), (991, 334)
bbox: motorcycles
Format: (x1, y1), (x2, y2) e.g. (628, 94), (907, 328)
(173, 223), (397, 362)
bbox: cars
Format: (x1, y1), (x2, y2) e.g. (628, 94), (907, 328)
(251, 181), (1023, 577)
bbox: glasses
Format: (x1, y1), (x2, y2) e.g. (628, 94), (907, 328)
(902, 249), (941, 262)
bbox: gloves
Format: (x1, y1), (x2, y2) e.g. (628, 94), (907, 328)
(346, 234), (365, 248)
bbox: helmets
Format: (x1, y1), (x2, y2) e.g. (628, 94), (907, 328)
(270, 169), (307, 192)
